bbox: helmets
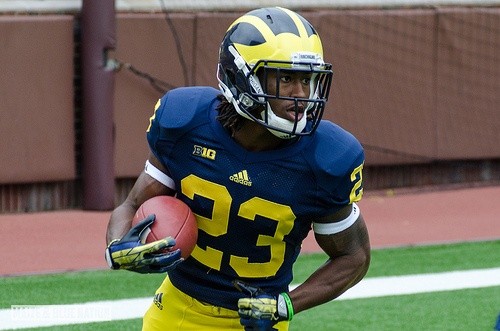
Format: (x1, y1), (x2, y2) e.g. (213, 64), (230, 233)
(214, 6), (332, 143)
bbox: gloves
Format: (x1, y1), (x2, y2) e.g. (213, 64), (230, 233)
(105, 213), (186, 273)
(236, 278), (293, 331)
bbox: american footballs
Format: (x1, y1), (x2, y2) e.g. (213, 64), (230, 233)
(132, 196), (198, 264)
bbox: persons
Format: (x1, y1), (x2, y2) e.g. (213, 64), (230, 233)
(105, 7), (370, 331)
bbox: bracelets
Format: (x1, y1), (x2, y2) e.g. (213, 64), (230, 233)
(282, 292), (294, 321)
